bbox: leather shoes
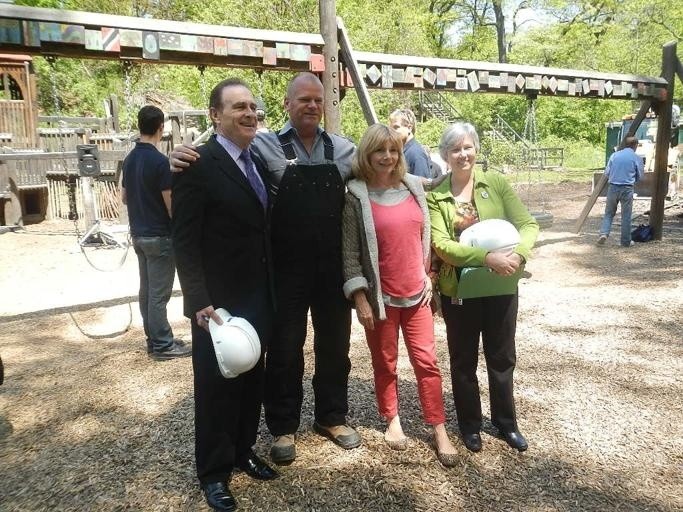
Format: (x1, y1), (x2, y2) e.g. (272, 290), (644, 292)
(203, 481), (238, 511)
(239, 451), (279, 481)
(464, 431), (481, 452)
(148, 340), (192, 361)
(502, 428), (528, 451)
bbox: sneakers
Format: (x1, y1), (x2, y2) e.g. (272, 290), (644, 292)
(438, 450), (460, 466)
(312, 418), (361, 448)
(271, 433), (297, 462)
(597, 234), (609, 244)
(385, 435), (408, 449)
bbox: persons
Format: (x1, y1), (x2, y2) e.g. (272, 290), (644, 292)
(425, 123), (539, 452)
(341, 124), (461, 467)
(121, 105), (192, 360)
(169, 71), (450, 512)
(597, 137), (644, 246)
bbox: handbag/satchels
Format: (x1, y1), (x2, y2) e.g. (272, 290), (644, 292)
(632, 224), (653, 242)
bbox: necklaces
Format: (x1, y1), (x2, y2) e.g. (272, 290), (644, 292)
(452, 173), (468, 201)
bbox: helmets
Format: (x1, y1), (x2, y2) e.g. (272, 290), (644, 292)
(459, 219), (520, 254)
(208, 308), (262, 379)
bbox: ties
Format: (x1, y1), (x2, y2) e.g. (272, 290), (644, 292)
(238, 148), (268, 212)
